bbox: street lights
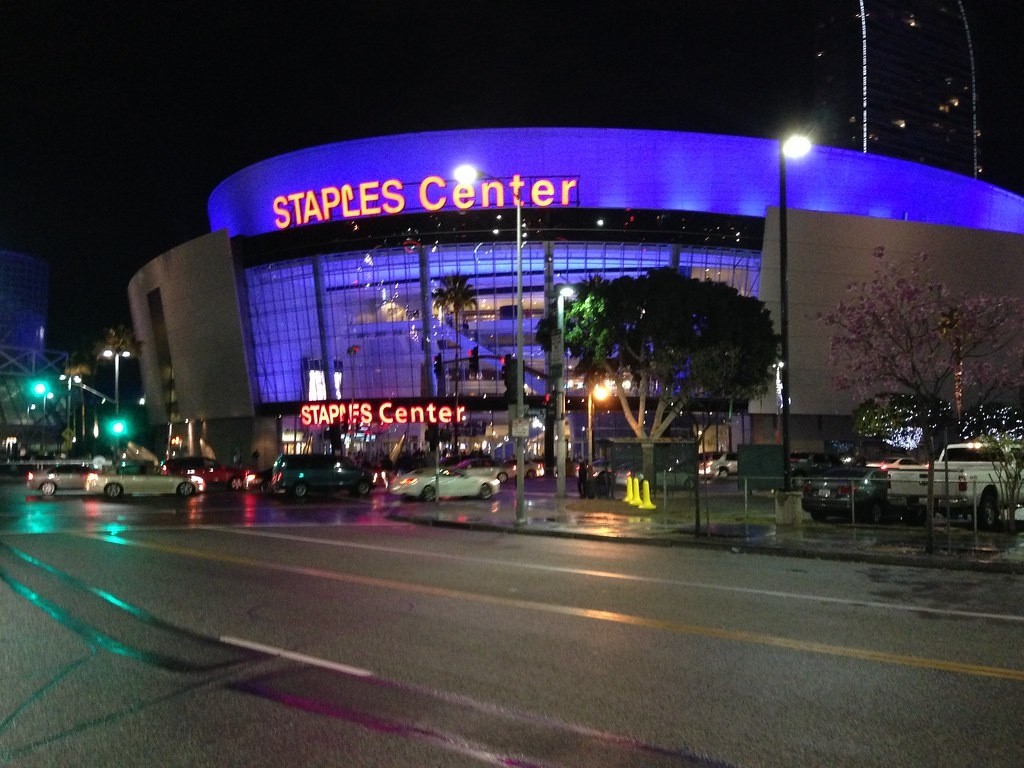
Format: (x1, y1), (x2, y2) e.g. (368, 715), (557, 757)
(59, 374), (81, 429)
(103, 349), (130, 455)
(453, 164), (524, 520)
(779, 133), (811, 494)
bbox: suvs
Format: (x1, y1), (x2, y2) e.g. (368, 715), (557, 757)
(161, 456), (249, 490)
(698, 451), (738, 477)
(272, 453), (375, 499)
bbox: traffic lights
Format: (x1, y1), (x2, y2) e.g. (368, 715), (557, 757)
(469, 347), (479, 374)
(501, 354), (509, 377)
(545, 393), (555, 419)
(434, 353), (442, 374)
(113, 421), (125, 434)
(34, 384), (46, 395)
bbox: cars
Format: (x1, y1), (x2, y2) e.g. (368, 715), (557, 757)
(577, 457), (691, 491)
(26, 463), (100, 495)
(245, 468), (272, 494)
(389, 457), (543, 502)
(791, 450), (925, 522)
(85, 464), (206, 498)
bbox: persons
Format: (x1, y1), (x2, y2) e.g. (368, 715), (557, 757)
(577, 456), (588, 499)
(347, 445), (495, 490)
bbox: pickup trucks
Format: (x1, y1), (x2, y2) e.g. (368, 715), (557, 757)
(885, 442), (1024, 529)
